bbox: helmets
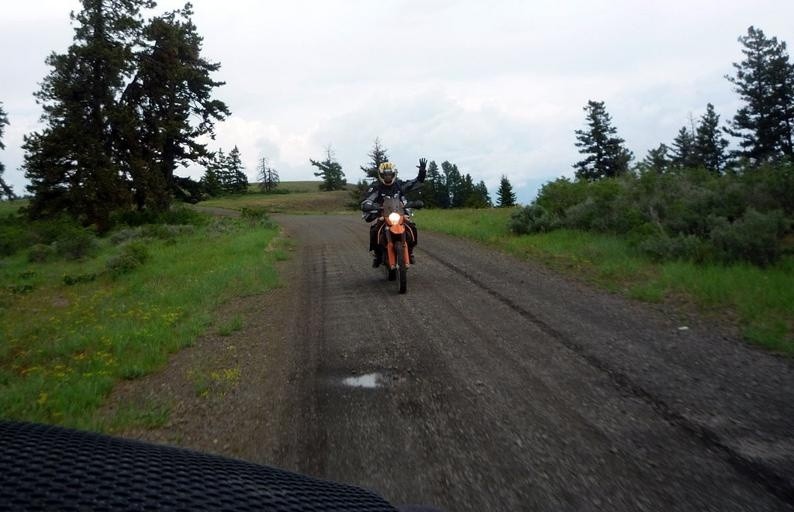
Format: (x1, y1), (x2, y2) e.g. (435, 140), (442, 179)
(378, 161), (398, 186)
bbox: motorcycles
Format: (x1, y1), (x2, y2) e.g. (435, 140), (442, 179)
(367, 198), (425, 294)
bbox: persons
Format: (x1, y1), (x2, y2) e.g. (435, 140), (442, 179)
(358, 157), (428, 268)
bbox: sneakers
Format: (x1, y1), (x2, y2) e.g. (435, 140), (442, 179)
(373, 255), (380, 267)
(410, 253), (416, 263)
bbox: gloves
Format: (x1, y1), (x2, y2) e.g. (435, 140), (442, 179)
(364, 202), (373, 209)
(416, 158), (428, 170)
